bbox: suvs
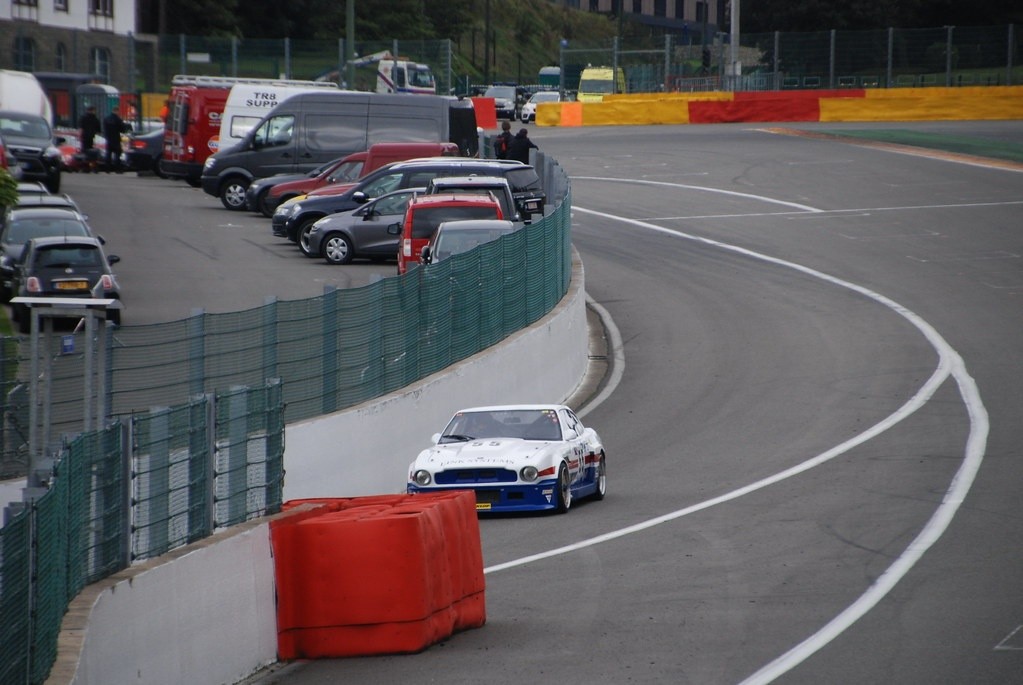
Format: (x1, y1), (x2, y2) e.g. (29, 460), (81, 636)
(483, 82), (528, 121)
(0, 142), (554, 336)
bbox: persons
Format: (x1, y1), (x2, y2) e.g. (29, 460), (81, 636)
(497, 121), (514, 159)
(79, 106), (100, 171)
(507, 128), (539, 166)
(104, 106), (133, 173)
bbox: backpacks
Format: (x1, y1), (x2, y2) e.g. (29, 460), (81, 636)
(494, 136), (509, 159)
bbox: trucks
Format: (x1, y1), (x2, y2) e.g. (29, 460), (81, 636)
(576, 65), (624, 103)
(317, 51), (437, 97)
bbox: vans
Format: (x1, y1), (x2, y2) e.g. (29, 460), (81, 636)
(0, 70), (481, 211)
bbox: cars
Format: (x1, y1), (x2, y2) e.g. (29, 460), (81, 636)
(406, 403), (606, 516)
(521, 92), (572, 125)
